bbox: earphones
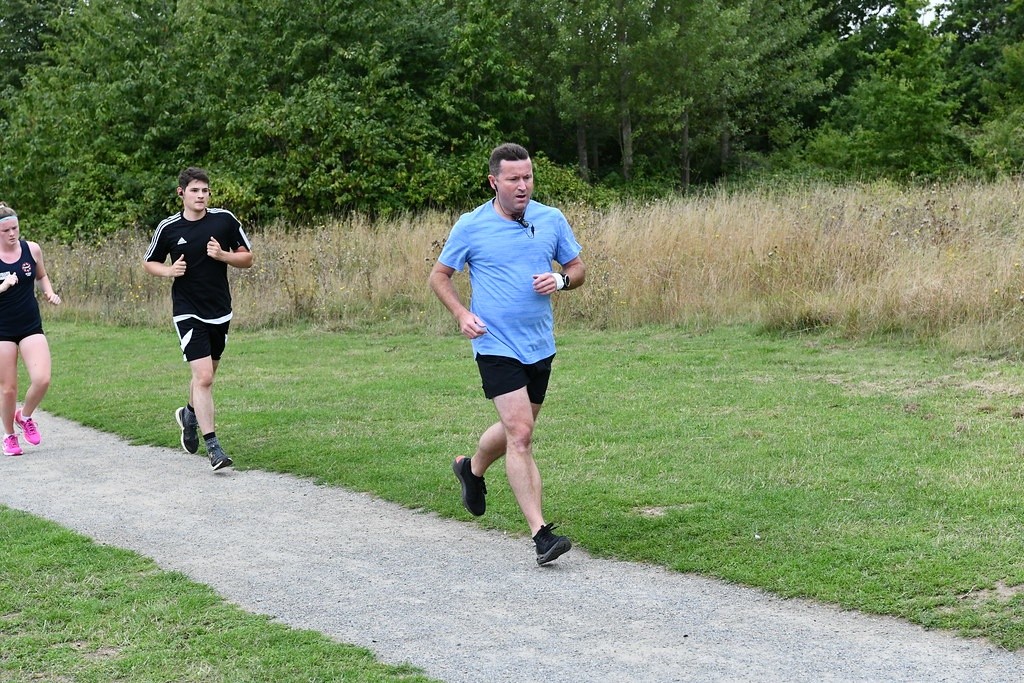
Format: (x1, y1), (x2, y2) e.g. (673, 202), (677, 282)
(179, 192), (183, 196)
(209, 193), (211, 196)
(493, 184), (498, 192)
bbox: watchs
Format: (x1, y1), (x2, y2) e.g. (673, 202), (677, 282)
(559, 273), (570, 290)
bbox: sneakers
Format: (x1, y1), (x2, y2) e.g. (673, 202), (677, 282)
(3, 435), (23, 456)
(535, 523), (572, 564)
(453, 455), (487, 516)
(15, 411), (40, 445)
(208, 448), (233, 471)
(175, 406), (199, 454)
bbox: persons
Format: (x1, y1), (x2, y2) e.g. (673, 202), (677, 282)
(0, 201), (61, 456)
(144, 169), (253, 470)
(428, 143), (583, 568)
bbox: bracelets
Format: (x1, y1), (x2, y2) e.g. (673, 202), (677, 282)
(551, 273), (564, 291)
